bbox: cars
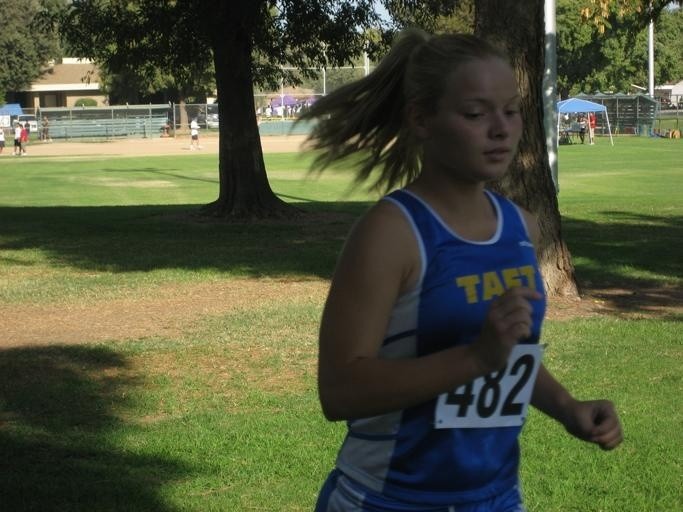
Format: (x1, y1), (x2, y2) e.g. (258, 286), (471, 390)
(198, 104), (220, 128)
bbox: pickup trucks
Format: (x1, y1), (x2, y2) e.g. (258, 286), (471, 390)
(12, 114), (37, 132)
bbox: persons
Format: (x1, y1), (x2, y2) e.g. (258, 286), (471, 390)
(189, 116), (203, 152)
(0, 117), (30, 158)
(41, 115), (51, 142)
(257, 101), (316, 120)
(576, 113), (587, 144)
(584, 111), (595, 145)
(287, 21), (627, 512)
